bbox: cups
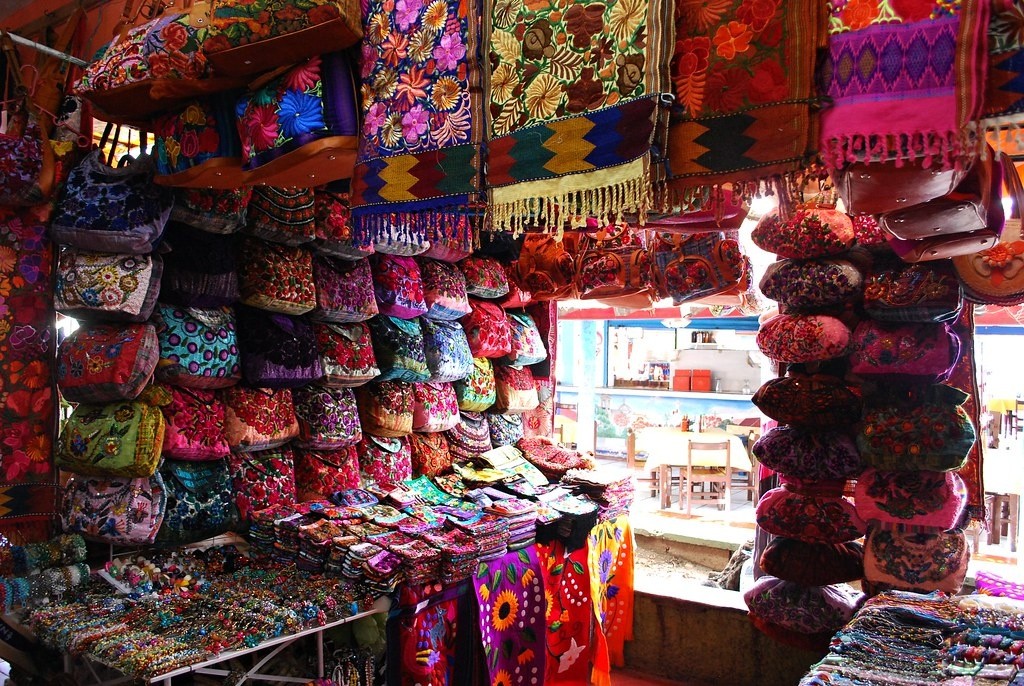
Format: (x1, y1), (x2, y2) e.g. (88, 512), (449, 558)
(682, 418), (689, 431)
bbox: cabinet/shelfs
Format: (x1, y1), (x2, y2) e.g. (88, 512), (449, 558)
(590, 315), (773, 476)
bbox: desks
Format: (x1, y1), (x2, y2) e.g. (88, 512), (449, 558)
(645, 426), (744, 511)
(5, 550), (395, 686)
(978, 446), (1023, 554)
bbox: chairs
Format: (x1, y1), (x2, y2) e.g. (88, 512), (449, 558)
(678, 439), (733, 519)
(711, 430), (762, 511)
(625, 427), (673, 509)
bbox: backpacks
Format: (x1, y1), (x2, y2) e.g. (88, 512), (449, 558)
(574, 219), (659, 308)
(509, 225), (577, 301)
(653, 224), (752, 305)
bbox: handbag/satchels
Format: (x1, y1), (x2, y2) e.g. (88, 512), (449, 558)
(743, 157), (1024, 651)
(53, 0), (548, 548)
